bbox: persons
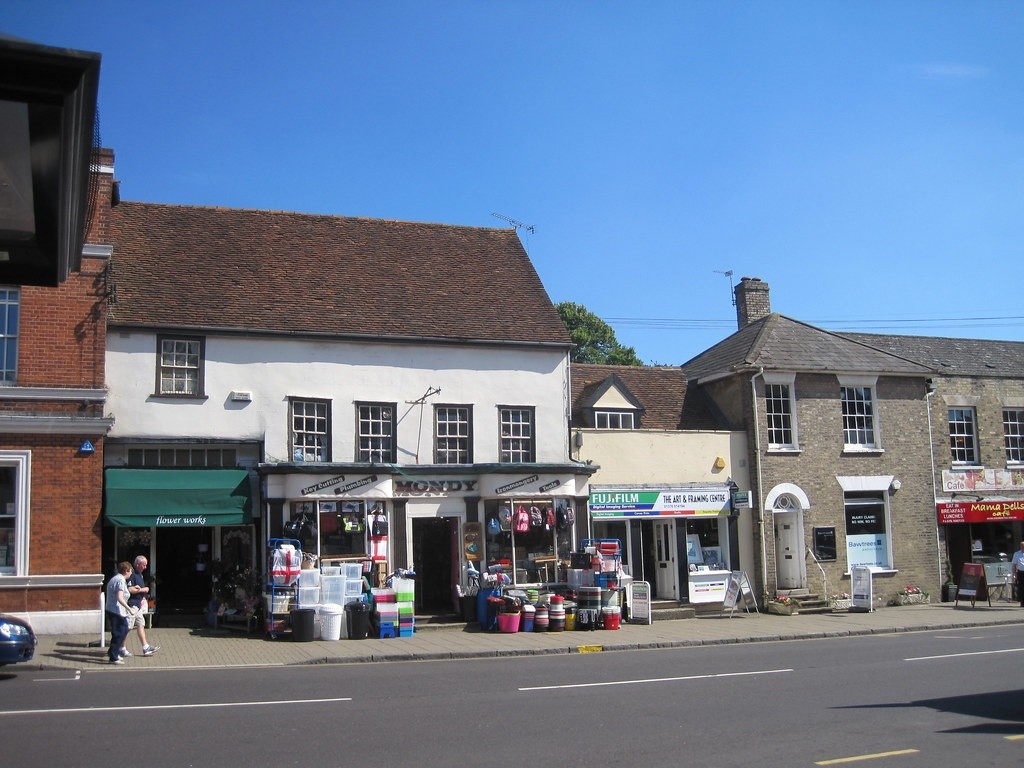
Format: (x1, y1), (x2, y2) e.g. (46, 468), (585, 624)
(122, 555), (160, 656)
(103, 560), (139, 666)
(1011, 541), (1024, 608)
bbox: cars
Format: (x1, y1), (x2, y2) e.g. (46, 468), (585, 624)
(0, 613), (38, 667)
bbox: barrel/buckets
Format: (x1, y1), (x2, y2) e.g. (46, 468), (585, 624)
(290, 609), (316, 642)
(344, 601), (371, 639)
(602, 606), (620, 630)
(320, 603), (343, 640)
(498, 612), (521, 633)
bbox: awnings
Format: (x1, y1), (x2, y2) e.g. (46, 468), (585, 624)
(102, 464), (254, 529)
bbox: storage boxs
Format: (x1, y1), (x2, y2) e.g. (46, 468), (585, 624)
(297, 561), (416, 640)
(467, 542), (626, 630)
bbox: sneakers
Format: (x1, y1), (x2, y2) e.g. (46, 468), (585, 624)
(119, 648), (132, 657)
(118, 654), (123, 660)
(143, 646), (161, 656)
(109, 659), (125, 665)
